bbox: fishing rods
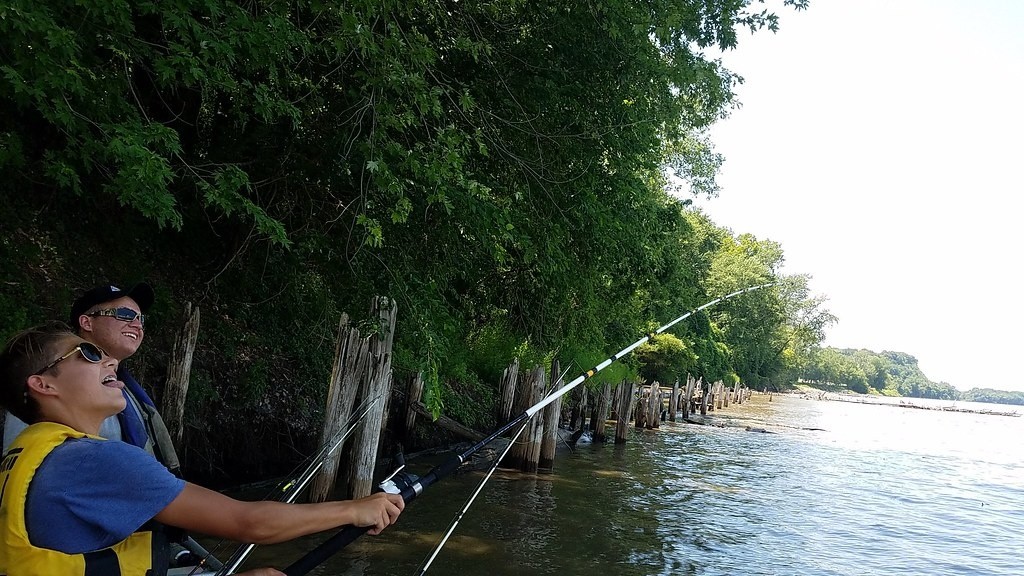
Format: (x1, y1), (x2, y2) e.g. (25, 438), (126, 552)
(287, 282), (776, 576)
(223, 388), (388, 575)
(415, 356), (575, 576)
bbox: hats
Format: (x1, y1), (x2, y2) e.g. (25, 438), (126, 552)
(70, 282), (155, 327)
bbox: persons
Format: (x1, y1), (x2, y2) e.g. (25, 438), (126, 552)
(2, 279), (185, 575)
(1, 320), (404, 576)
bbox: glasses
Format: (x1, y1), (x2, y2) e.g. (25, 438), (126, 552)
(84, 307), (145, 325)
(23, 342), (110, 404)
